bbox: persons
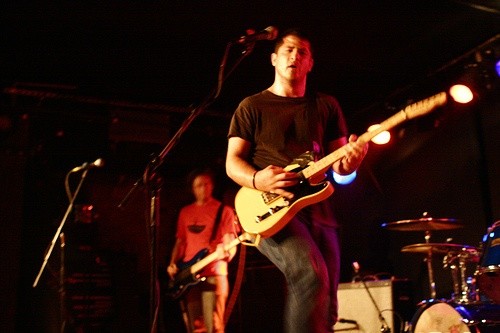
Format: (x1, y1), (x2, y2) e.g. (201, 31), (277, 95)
(226, 27), (369, 333)
(167, 169), (240, 333)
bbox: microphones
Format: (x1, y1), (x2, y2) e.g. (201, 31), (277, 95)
(236, 26), (278, 45)
(72, 159), (104, 173)
(339, 319), (357, 325)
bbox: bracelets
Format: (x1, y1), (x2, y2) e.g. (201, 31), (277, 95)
(253, 170), (259, 190)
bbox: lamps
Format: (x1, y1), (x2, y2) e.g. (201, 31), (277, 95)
(446, 66), (483, 106)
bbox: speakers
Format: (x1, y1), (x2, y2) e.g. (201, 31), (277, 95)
(333, 279), (413, 333)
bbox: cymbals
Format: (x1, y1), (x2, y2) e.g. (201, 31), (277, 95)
(400, 242), (469, 255)
(380, 214), (468, 233)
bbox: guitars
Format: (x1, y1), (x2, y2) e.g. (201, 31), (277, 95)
(234, 90), (449, 238)
(167, 234), (249, 295)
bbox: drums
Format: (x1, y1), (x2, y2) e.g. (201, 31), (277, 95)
(475, 219), (500, 305)
(400, 298), (483, 333)
(438, 250), (482, 304)
